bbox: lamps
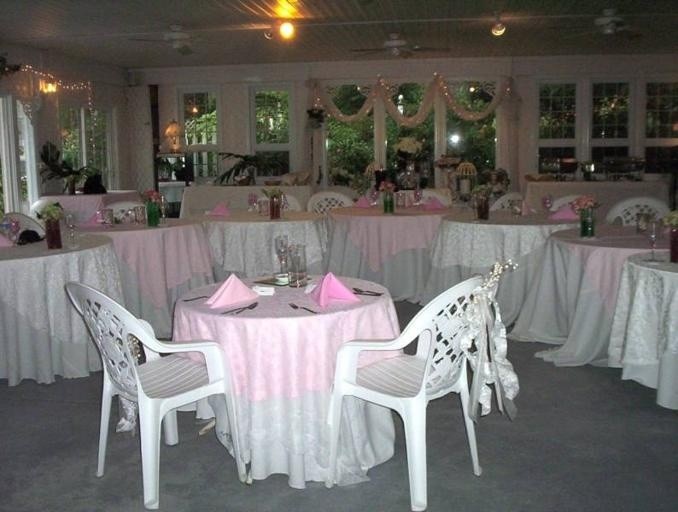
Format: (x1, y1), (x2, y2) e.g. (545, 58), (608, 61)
(163, 118), (182, 154)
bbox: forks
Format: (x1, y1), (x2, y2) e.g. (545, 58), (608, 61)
(352, 287), (385, 295)
(288, 303), (317, 314)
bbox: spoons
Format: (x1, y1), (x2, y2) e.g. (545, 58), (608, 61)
(235, 301), (259, 314)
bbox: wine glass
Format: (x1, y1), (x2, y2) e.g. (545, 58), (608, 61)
(157, 193), (169, 226)
(275, 236), (291, 280)
(644, 221), (663, 262)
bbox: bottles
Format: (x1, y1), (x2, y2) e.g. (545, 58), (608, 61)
(269, 193), (289, 222)
(400, 163), (421, 211)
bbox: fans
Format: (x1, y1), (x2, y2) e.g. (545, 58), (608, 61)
(128, 25), (213, 56)
(548, 8), (646, 42)
(349, 32), (443, 57)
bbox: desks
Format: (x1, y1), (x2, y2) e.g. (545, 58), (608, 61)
(521, 173), (671, 226)
(179, 185), (315, 219)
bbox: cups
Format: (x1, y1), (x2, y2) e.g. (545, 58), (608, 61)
(286, 246), (307, 288)
